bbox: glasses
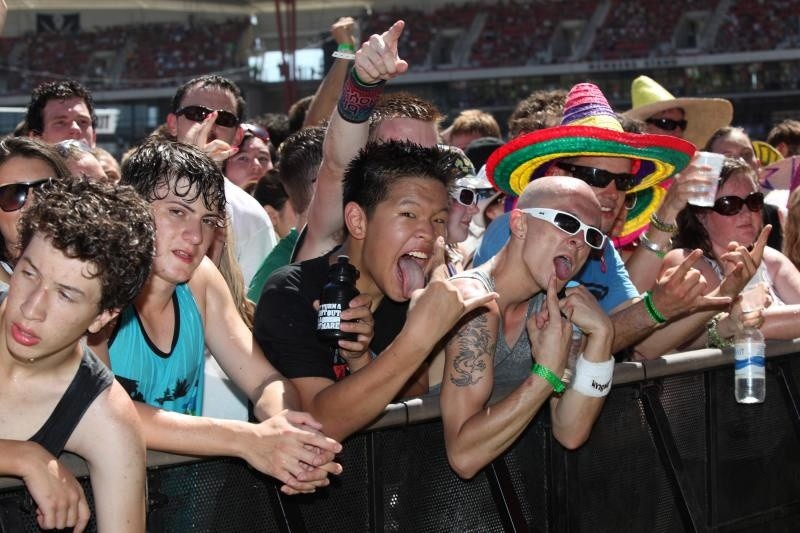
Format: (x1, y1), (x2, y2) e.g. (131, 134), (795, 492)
(240, 123), (270, 142)
(623, 195), (638, 210)
(704, 192), (765, 217)
(555, 160), (636, 192)
(0, 178), (60, 211)
(519, 207), (605, 249)
(644, 116), (689, 132)
(176, 105), (239, 128)
(448, 187), (479, 206)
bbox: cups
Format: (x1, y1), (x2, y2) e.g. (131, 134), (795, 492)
(687, 151), (727, 208)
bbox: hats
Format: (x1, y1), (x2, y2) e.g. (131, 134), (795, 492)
(749, 140), (784, 167)
(437, 145), (492, 189)
(463, 136), (504, 179)
(755, 156), (800, 196)
(486, 83), (696, 197)
(606, 176), (677, 246)
(618, 76), (734, 151)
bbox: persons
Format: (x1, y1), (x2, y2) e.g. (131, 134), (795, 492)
(251, 137), (500, 444)
(89, 139), (341, 496)
(1, 0), (800, 346)
(0, 174), (157, 533)
(474, 82), (772, 369)
(430, 176), (615, 487)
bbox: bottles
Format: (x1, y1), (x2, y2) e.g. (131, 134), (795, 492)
(734, 325), (766, 404)
(316, 255), (360, 345)
(560, 280), (583, 387)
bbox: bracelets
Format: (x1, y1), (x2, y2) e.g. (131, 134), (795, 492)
(573, 353), (614, 397)
(639, 231), (672, 257)
(643, 293), (667, 324)
(530, 363), (565, 392)
(708, 319), (732, 347)
(337, 43), (354, 52)
(336, 63), (386, 124)
(650, 213), (678, 233)
